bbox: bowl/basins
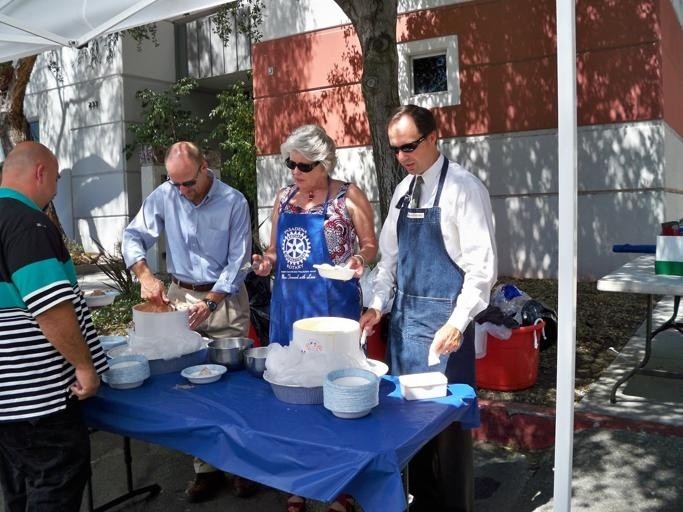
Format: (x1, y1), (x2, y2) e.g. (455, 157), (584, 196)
(98, 335), (213, 390)
(179, 363), (227, 385)
(205, 337), (254, 370)
(311, 264), (358, 282)
(243, 347), (267, 378)
(322, 368), (379, 417)
(291, 317), (360, 355)
(82, 291), (120, 307)
(262, 357), (389, 405)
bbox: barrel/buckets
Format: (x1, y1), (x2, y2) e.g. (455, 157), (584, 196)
(362, 308), (388, 361)
(475, 318), (546, 391)
(291, 316), (362, 371)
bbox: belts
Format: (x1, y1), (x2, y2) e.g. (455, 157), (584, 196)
(173, 278), (215, 291)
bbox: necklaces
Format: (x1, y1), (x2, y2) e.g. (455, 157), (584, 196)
(308, 191), (314, 201)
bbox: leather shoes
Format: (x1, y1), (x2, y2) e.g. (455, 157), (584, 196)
(232, 475), (258, 497)
(185, 470), (224, 502)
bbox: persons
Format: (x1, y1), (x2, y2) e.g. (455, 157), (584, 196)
(0, 140), (111, 512)
(358, 103), (500, 512)
(250, 124), (379, 511)
(120, 140), (252, 503)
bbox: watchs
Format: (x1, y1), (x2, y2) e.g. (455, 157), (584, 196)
(202, 298), (217, 313)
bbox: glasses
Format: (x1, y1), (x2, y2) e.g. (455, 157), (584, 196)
(391, 135), (425, 154)
(172, 165), (200, 186)
(286, 157), (319, 172)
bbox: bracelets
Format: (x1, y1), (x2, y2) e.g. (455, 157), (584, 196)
(354, 254), (365, 266)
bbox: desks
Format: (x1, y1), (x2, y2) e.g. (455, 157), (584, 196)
(593, 252), (682, 404)
(75, 343), (481, 512)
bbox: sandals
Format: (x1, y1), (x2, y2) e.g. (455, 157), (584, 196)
(327, 494), (354, 512)
(288, 495), (305, 512)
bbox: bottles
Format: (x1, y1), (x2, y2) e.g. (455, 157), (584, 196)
(661, 218), (682, 235)
